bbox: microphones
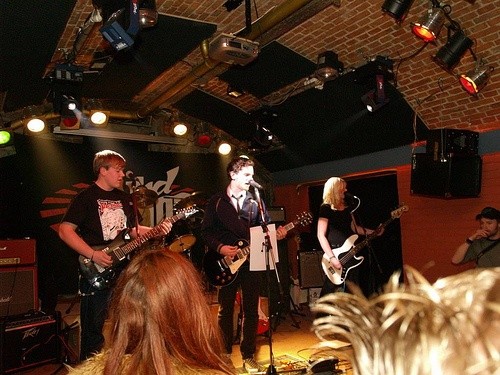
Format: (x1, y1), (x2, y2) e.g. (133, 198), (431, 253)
(342, 191), (360, 202)
(247, 178), (263, 189)
(126, 170), (140, 183)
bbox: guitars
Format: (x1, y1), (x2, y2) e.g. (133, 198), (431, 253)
(235, 286), (270, 337)
(321, 205), (409, 285)
(76, 205), (199, 294)
(203, 211), (314, 287)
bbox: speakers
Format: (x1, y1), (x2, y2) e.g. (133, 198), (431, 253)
(409, 128), (481, 200)
(297, 248), (325, 288)
(265, 206), (286, 221)
(0, 265), (38, 319)
(0, 318), (59, 375)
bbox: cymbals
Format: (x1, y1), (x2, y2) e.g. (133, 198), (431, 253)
(129, 188), (158, 208)
(173, 191), (211, 216)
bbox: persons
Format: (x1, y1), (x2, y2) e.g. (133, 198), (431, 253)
(59, 149), (172, 363)
(452, 207), (500, 267)
(201, 156), (287, 373)
(316, 177), (384, 325)
(64, 248), (235, 375)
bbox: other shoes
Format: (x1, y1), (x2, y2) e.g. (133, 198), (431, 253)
(242, 357), (259, 369)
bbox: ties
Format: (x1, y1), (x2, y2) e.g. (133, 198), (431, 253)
(232, 194), (243, 214)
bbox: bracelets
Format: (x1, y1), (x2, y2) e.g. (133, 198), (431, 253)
(219, 245), (224, 254)
(464, 237), (474, 244)
(91, 251), (94, 260)
(329, 256), (335, 259)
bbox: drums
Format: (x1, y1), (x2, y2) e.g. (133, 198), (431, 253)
(164, 219), (197, 253)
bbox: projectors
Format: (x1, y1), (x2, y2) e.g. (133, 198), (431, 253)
(209, 35), (259, 67)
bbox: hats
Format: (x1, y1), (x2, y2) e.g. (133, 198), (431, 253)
(475, 207), (500, 220)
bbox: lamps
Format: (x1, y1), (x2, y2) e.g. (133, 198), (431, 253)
(138, 0), (159, 29)
(0, 62), (231, 156)
(316, 51), (394, 114)
(382, 0), (495, 97)
